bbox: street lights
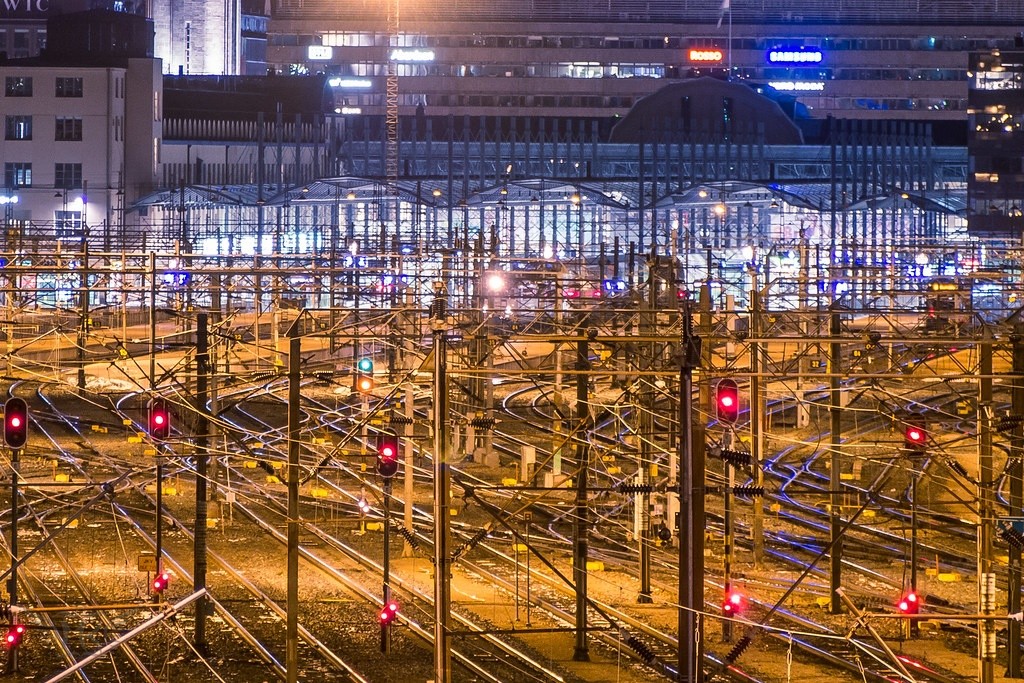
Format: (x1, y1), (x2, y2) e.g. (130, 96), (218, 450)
(1008, 207), (1022, 283)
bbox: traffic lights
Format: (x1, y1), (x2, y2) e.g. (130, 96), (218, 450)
(6, 397), (29, 447)
(357, 359), (376, 394)
(153, 573), (171, 594)
(147, 397), (171, 446)
(903, 411), (926, 456)
(375, 427), (401, 479)
(380, 600), (400, 624)
(715, 377), (739, 428)
(6, 624), (23, 647)
(899, 593), (920, 615)
(721, 593), (742, 614)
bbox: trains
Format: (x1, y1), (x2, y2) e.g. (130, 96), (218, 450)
(922, 275), (1024, 332)
